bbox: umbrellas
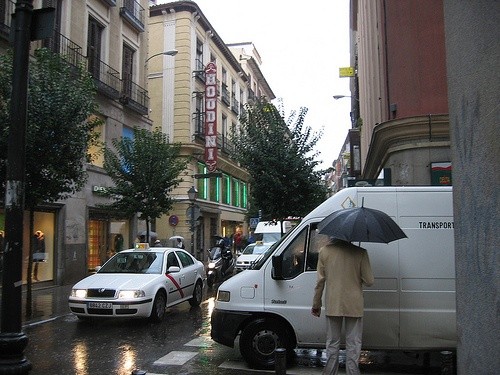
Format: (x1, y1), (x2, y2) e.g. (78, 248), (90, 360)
(169, 236), (185, 241)
(315, 197), (409, 249)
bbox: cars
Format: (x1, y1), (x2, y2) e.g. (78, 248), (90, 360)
(67, 242), (208, 324)
(236, 240), (275, 273)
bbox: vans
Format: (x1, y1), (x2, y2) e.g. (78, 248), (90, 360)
(254, 221), (292, 243)
(210, 185), (456, 372)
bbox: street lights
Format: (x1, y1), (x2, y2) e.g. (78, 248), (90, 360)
(188, 185), (199, 259)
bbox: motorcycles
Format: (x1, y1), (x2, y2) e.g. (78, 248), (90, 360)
(206, 247), (235, 289)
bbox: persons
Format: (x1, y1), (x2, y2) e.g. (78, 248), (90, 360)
(176, 239), (183, 249)
(132, 230), (162, 248)
(113, 231), (123, 252)
(32, 230), (46, 282)
(211, 231), (244, 252)
(311, 238), (374, 374)
